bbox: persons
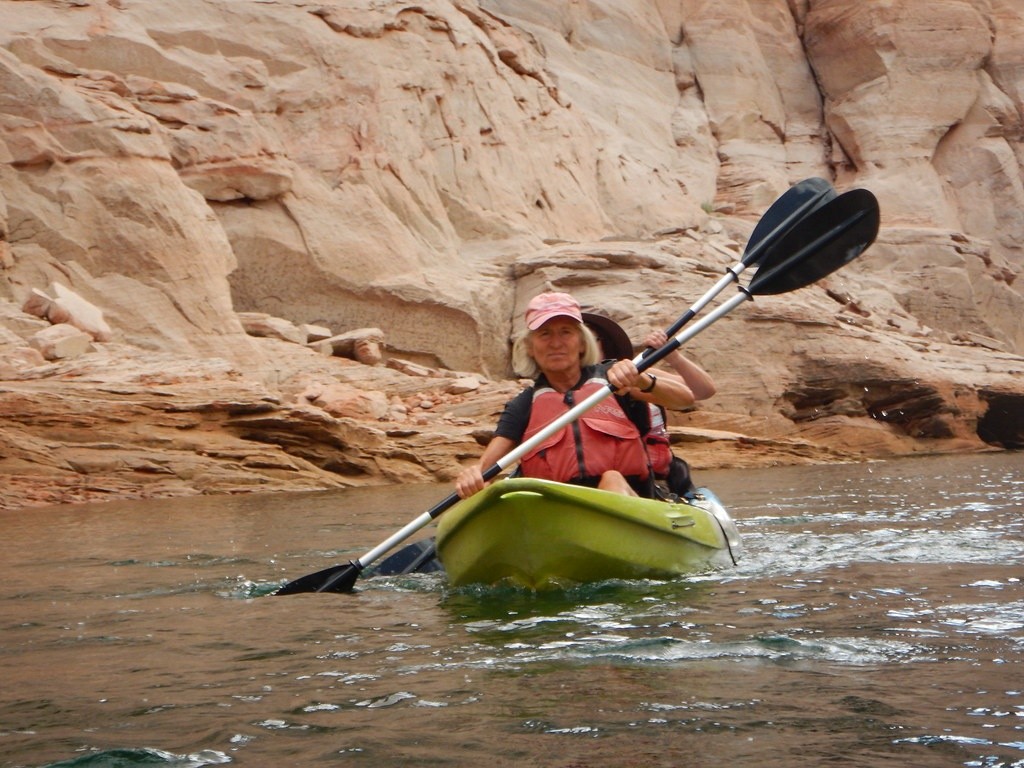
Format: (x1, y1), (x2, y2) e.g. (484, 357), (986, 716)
(576, 310), (718, 494)
(454, 292), (694, 501)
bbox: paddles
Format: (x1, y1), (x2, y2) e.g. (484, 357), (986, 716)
(271, 187), (882, 597)
(358, 177), (837, 580)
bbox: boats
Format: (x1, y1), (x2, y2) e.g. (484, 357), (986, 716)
(434, 477), (745, 600)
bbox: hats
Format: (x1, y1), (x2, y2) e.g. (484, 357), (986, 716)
(580, 313), (634, 362)
(525, 291), (584, 331)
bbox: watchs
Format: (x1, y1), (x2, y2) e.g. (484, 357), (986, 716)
(639, 372), (657, 393)
